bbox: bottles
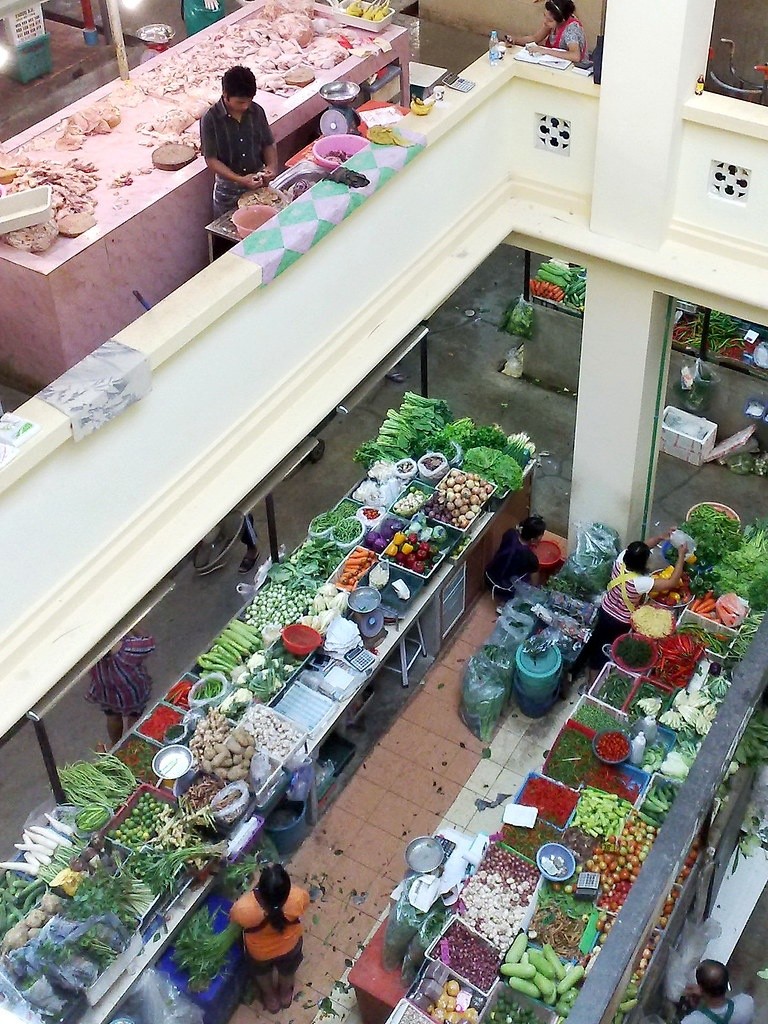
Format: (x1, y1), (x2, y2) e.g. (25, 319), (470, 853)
(695, 75), (705, 95)
(644, 715), (656, 726)
(630, 732), (646, 763)
(489, 31), (499, 65)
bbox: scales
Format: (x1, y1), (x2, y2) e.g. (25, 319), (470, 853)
(346, 585), (389, 649)
(318, 81), (362, 137)
(151, 744), (196, 798)
(403, 837), (444, 880)
(136, 24), (176, 65)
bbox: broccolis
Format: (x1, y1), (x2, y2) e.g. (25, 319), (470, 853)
(221, 654), (296, 717)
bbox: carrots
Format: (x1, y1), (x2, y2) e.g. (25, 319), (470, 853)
(530, 279), (564, 301)
(692, 590), (745, 628)
(336, 548), (375, 588)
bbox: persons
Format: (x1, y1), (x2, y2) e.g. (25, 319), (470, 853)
(386, 370), (407, 385)
(579, 527), (690, 695)
(239, 519), (261, 574)
(506, 0), (589, 69)
(678, 959), (756, 1024)
(86, 625), (157, 755)
(200, 65), (276, 252)
(230, 865), (310, 1014)
(486, 517), (545, 601)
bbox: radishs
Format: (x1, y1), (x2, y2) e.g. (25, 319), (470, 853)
(0, 812), (85, 882)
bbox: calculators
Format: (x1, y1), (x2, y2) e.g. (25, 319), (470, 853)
(574, 872), (601, 901)
(344, 646), (376, 673)
(433, 835), (456, 865)
(442, 72), (476, 93)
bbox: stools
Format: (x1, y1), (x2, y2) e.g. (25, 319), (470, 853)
(407, 60), (447, 101)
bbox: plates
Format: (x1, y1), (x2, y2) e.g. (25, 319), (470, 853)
(648, 569), (696, 607)
(630, 606), (676, 638)
(612, 634), (658, 672)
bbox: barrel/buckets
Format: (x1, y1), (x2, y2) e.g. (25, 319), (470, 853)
(514, 674), (559, 719)
(264, 801), (307, 854)
(515, 642), (561, 689)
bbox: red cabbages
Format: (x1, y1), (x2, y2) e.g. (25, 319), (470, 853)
(366, 519), (402, 549)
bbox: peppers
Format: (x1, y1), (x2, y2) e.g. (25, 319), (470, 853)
(385, 531), (438, 573)
(660, 636), (701, 688)
(649, 565), (690, 605)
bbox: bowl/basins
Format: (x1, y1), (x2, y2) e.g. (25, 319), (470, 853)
(312, 134), (370, 169)
(232, 205), (279, 238)
(534, 541), (561, 569)
(686, 502), (740, 533)
(347, 586), (381, 613)
(282, 625), (320, 655)
(591, 728), (632, 763)
(404, 836), (445, 873)
(319, 81), (361, 106)
(152, 745), (193, 779)
(662, 541), (713, 575)
(136, 24), (176, 45)
(536, 843), (576, 881)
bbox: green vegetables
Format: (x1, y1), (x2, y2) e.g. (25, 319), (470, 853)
(353, 392), (535, 491)
(678, 520), (768, 610)
(69, 847), (261, 983)
(290, 540), (345, 576)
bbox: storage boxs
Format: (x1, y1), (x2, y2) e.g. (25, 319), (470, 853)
(0, 3), (53, 85)
(0, 185), (52, 236)
(657, 405), (718, 466)
(335, 0), (395, 32)
(0, 455), (754, 1022)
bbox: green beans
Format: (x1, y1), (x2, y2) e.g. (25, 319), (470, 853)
(58, 753), (136, 823)
(311, 503), (362, 544)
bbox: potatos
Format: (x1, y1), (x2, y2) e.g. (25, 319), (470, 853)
(201, 731), (254, 782)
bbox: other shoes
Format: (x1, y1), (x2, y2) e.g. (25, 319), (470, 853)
(387, 372), (403, 382)
(256, 980), (295, 1014)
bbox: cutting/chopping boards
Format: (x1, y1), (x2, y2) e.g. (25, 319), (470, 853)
(152, 144), (196, 171)
(238, 189), (288, 211)
(285, 66), (315, 88)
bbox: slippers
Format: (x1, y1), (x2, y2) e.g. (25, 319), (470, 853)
(195, 558), (227, 575)
(238, 550), (262, 574)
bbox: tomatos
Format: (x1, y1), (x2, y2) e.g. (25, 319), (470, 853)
(554, 818), (657, 932)
